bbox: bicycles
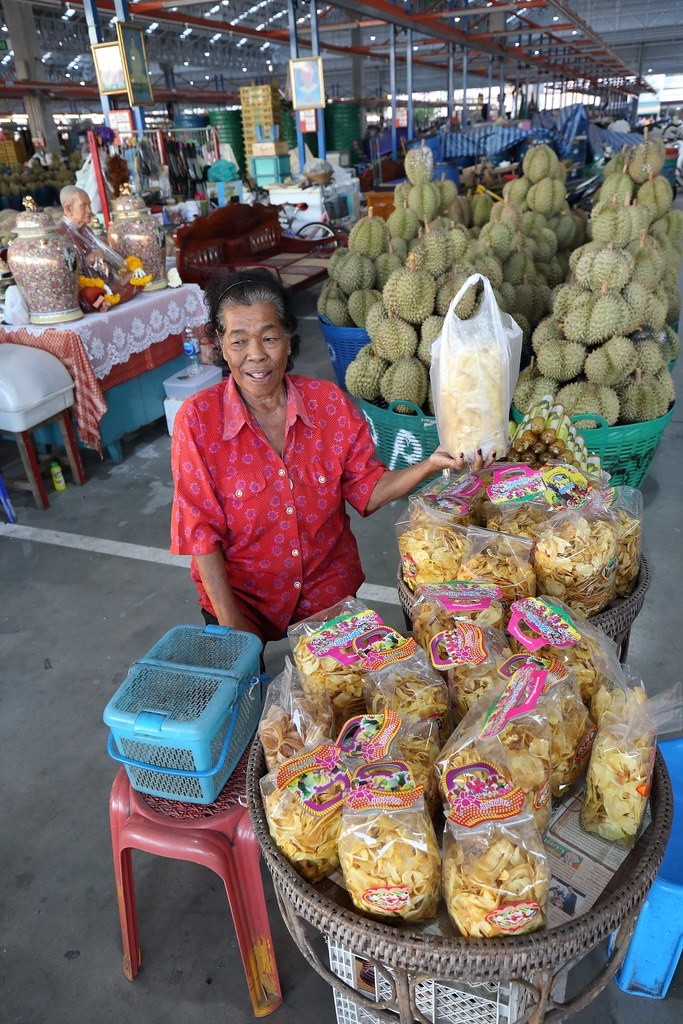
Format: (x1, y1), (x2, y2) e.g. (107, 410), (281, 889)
(243, 178), (338, 258)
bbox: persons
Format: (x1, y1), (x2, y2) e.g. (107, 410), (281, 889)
(59, 185), (110, 277)
(607, 116), (631, 134)
(634, 115), (656, 127)
(171, 268), (498, 674)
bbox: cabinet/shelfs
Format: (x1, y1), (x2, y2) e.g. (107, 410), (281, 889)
(367, 192), (396, 223)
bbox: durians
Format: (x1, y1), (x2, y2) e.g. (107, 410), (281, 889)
(317, 126), (683, 431)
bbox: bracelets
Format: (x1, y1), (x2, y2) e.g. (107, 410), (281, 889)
(81, 256), (86, 272)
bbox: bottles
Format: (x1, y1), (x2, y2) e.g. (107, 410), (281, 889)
(183, 327), (204, 376)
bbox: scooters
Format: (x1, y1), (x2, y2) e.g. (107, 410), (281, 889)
(473, 118), (683, 220)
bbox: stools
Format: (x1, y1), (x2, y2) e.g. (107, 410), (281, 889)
(607, 738), (683, 999)
(108, 730), (284, 1017)
(0, 472), (17, 525)
(0, 408), (86, 510)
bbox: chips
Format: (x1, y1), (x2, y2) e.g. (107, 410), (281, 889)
(255, 474), (655, 939)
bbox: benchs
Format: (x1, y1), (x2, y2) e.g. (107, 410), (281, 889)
(174, 203), (349, 300)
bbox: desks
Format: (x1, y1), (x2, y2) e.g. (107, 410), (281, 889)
(1, 281), (217, 464)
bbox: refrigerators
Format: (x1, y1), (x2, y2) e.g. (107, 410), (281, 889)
(267, 178), (361, 238)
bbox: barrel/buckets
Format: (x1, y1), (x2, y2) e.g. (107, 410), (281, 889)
(277, 106), (320, 159)
(176, 115), (207, 126)
(210, 111), (248, 178)
(324, 102), (369, 164)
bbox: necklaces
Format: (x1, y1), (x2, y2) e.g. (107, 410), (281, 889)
(247, 384), (294, 491)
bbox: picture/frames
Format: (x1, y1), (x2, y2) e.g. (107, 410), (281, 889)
(289, 56), (325, 111)
(116, 21), (154, 107)
(91, 41), (128, 95)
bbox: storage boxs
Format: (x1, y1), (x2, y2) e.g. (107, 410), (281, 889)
(163, 364), (223, 398)
(663, 148), (680, 186)
(164, 396), (185, 437)
(171, 80), (366, 187)
(0, 131), (29, 167)
(327, 935), (571, 1024)
